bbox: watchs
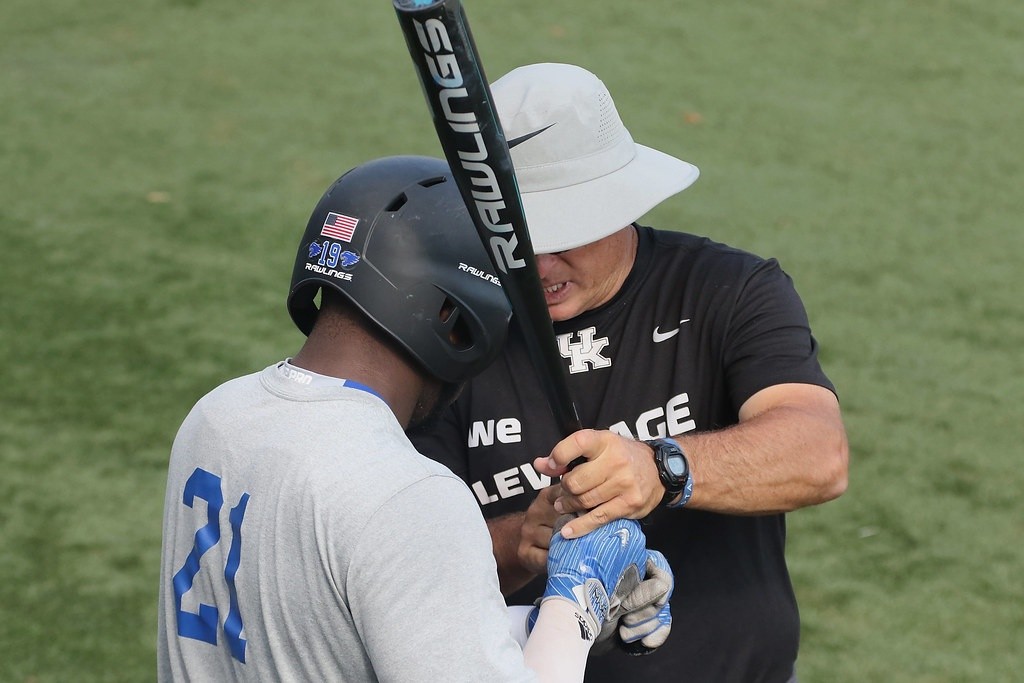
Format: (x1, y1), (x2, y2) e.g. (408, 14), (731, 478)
(644, 439), (689, 513)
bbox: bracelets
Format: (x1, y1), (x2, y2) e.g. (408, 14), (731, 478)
(658, 437), (694, 510)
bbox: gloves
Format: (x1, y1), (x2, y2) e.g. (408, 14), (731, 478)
(526, 549), (672, 649)
(541, 511), (646, 629)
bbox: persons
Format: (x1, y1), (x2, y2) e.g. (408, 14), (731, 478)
(403, 64), (848, 683)
(157, 153), (675, 683)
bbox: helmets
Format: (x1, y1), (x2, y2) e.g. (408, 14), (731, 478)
(287, 155), (514, 383)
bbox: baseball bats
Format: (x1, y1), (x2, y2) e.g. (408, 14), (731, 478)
(393, 0), (585, 470)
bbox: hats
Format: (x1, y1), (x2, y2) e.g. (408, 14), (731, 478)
(487, 63), (700, 254)
